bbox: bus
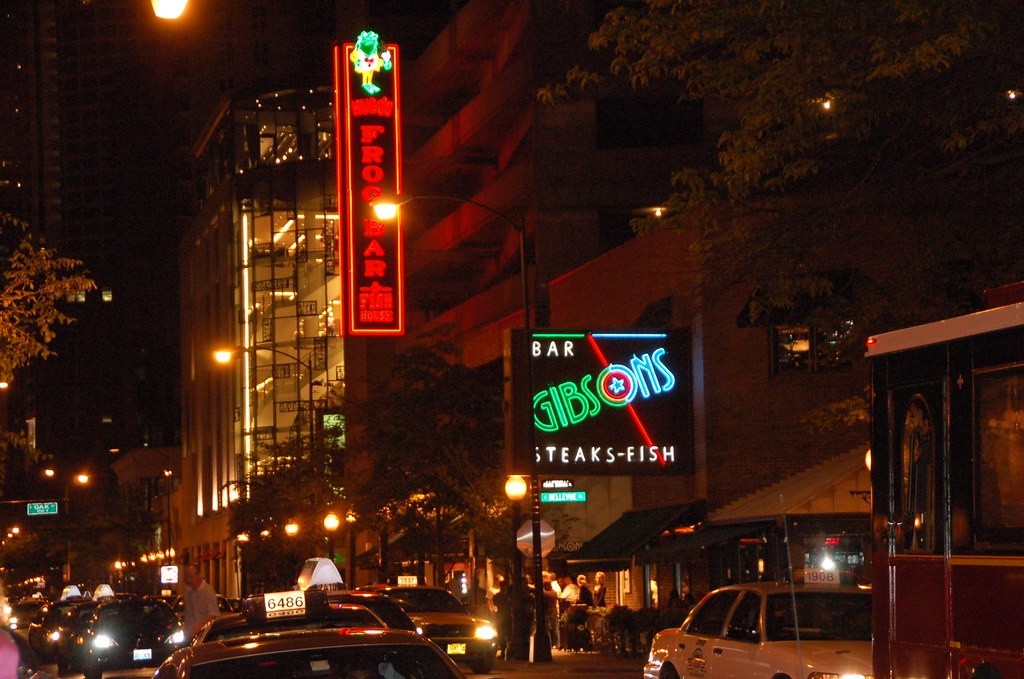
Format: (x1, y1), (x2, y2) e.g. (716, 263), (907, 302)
(860, 280), (1024, 679)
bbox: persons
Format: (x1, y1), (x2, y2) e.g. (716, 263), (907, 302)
(811, 596), (873, 640)
(448, 572), (703, 660)
(181, 563), (218, 640)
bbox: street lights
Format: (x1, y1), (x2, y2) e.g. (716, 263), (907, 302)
(283, 518), (302, 588)
(43, 468), (91, 585)
(235, 530), (250, 598)
(325, 512), (339, 563)
(213, 347), (318, 563)
(503, 477), (527, 664)
(370, 192), (557, 664)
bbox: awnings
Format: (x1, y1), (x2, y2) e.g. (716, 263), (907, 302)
(199, 549), (209, 561)
(564, 500), (694, 570)
(177, 549), (188, 561)
(215, 545), (225, 559)
(208, 548), (217, 560)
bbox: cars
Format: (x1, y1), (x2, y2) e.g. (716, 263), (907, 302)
(146, 585), (472, 679)
(373, 584), (501, 675)
(642, 568), (874, 679)
(5, 583), (134, 676)
(76, 584), (186, 679)
